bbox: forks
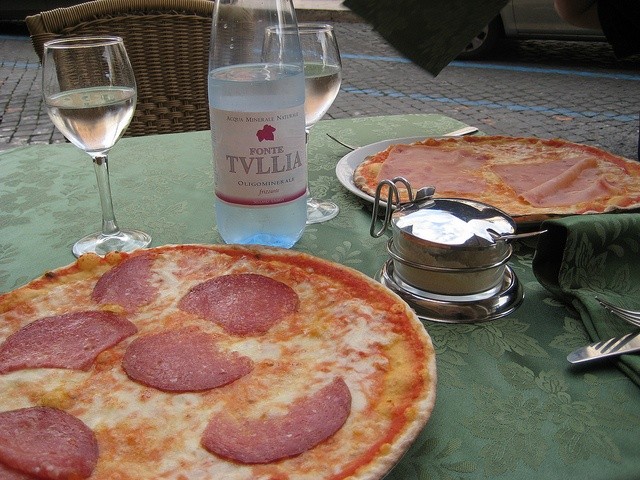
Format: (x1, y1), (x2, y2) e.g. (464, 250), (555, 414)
(323, 126), (484, 152)
(595, 295), (639, 327)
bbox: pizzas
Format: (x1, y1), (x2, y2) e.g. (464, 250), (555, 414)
(1, 244), (437, 480)
(356, 137), (640, 216)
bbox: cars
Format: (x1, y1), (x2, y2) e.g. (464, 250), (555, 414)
(451, 0), (610, 61)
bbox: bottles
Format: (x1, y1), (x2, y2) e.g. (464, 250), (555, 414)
(206, 0), (307, 249)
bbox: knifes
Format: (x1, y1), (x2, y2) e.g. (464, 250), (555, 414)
(567, 329), (640, 362)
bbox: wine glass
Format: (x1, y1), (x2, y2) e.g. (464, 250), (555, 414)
(42, 35), (152, 259)
(261, 24), (341, 224)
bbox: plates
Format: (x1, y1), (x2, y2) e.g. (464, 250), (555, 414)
(333, 135), (463, 212)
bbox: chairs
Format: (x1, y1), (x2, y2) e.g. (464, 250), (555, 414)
(25, 0), (255, 143)
